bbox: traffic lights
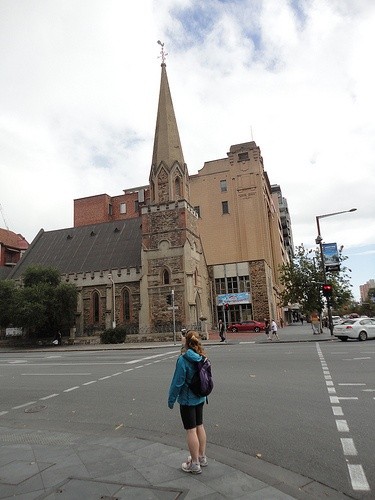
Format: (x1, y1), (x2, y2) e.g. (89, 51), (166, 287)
(322, 284), (333, 296)
(225, 304), (229, 310)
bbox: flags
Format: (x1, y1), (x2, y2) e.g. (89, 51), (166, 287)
(322, 242), (340, 271)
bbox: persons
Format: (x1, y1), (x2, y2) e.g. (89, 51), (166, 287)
(181, 329), (188, 355)
(168, 330), (210, 474)
(300, 318), (303, 325)
(280, 318), (284, 328)
(270, 319), (280, 340)
(324, 317), (328, 327)
(264, 317), (270, 339)
(350, 315), (359, 319)
(219, 320), (225, 342)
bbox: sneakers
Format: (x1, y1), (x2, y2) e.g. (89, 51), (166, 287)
(188, 455), (208, 466)
(182, 461), (202, 474)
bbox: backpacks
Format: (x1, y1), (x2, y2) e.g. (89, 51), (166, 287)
(181, 352), (213, 404)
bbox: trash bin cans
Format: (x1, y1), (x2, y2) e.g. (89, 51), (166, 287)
(313, 321), (322, 334)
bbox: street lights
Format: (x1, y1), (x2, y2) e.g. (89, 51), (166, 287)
(315, 208), (358, 335)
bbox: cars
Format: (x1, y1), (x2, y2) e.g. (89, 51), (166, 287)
(318, 313), (368, 327)
(227, 320), (266, 333)
(332, 317), (375, 341)
